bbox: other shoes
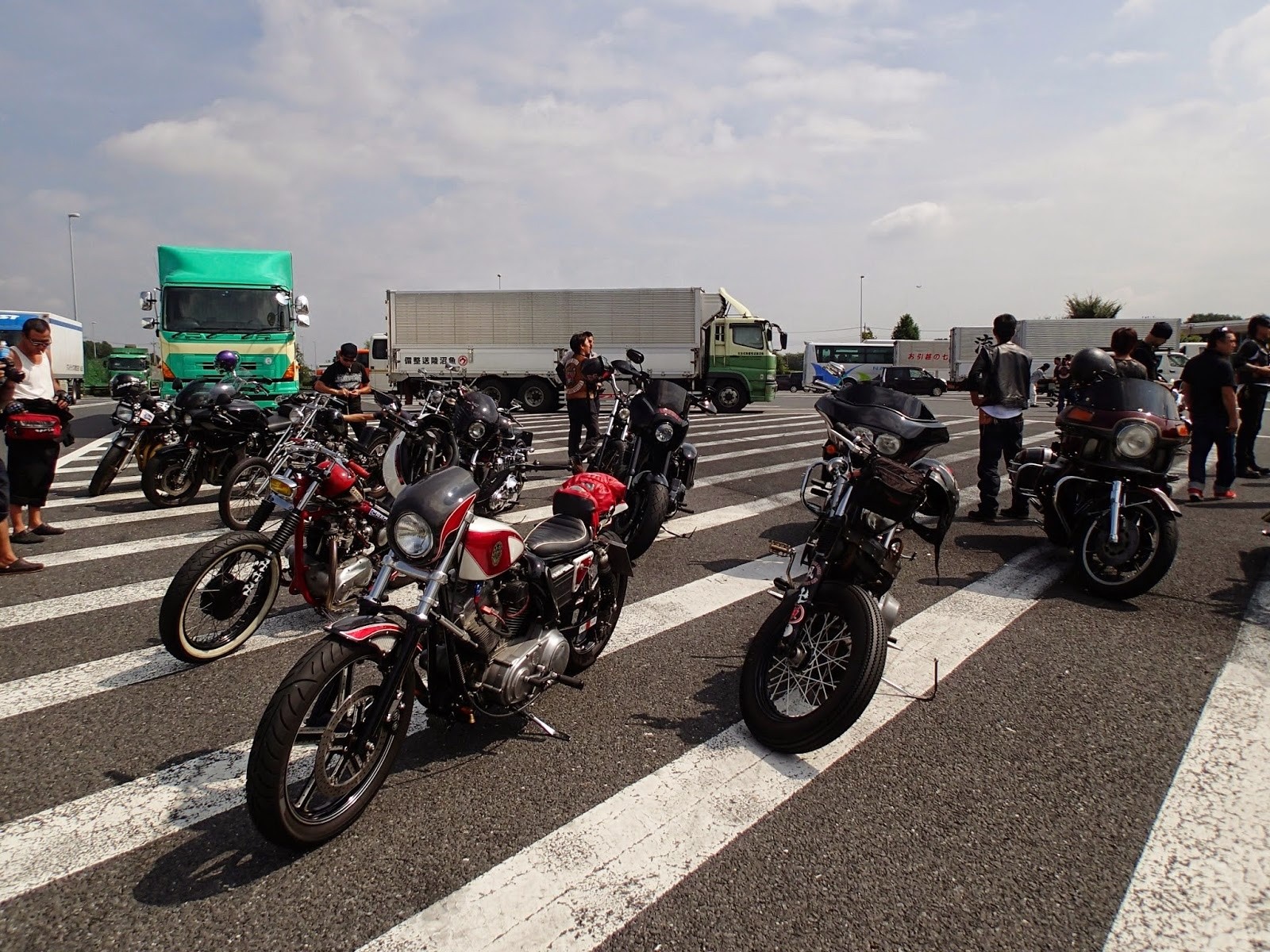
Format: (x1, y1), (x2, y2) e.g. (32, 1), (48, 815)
(576, 441), (597, 456)
(1000, 508), (1030, 518)
(1046, 402), (1053, 407)
(1, 557), (45, 574)
(1187, 487), (1204, 501)
(1030, 404), (1039, 408)
(1249, 462), (1270, 474)
(568, 456), (584, 476)
(1213, 487), (1237, 499)
(1236, 466), (1261, 479)
(967, 510), (997, 521)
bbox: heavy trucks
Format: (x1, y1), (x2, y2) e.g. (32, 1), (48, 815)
(384, 285), (789, 415)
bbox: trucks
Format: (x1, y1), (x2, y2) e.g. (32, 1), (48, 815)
(102, 344), (162, 396)
(139, 241), (311, 412)
(0, 310), (85, 405)
(857, 316), (1208, 395)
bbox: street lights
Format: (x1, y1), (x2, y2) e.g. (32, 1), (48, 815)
(68, 213), (80, 321)
(91, 321), (97, 359)
(497, 273), (501, 289)
(859, 275), (866, 343)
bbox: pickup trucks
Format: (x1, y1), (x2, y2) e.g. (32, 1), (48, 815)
(860, 366), (949, 397)
(776, 371), (804, 393)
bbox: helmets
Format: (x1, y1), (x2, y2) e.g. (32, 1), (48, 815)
(1070, 347), (1118, 381)
(109, 374), (150, 397)
(214, 350), (241, 373)
(581, 356), (614, 380)
(909, 457), (960, 546)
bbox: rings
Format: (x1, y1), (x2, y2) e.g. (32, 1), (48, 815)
(344, 392), (346, 394)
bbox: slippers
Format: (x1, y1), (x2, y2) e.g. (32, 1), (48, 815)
(9, 530), (45, 544)
(32, 523), (65, 535)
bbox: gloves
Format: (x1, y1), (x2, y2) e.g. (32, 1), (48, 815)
(330, 411), (345, 424)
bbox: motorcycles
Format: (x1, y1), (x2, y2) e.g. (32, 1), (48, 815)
(87, 346), (720, 852)
(737, 359), (951, 755)
(1008, 373), (1190, 601)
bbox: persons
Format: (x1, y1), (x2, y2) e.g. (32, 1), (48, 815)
(1, 317), (72, 573)
(316, 342), (370, 441)
(556, 331), (609, 472)
(968, 313), (1270, 521)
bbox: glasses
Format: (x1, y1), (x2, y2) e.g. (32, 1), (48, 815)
(29, 338), (52, 347)
(342, 358), (354, 362)
(1219, 326), (1229, 333)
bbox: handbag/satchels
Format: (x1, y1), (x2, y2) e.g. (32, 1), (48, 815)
(5, 412), (64, 443)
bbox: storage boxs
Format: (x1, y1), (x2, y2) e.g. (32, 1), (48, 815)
(1008, 447), (1056, 492)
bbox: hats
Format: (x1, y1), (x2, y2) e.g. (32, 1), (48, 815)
(1150, 322), (1172, 339)
(340, 342), (358, 358)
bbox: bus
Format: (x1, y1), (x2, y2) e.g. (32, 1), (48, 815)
(801, 341), (894, 394)
